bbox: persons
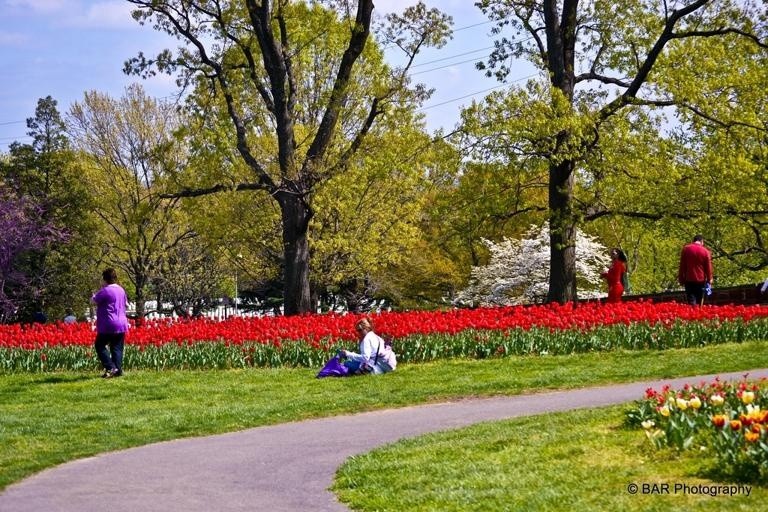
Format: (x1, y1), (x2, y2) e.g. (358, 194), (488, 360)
(90, 268), (129, 378)
(32, 308), (47, 324)
(599, 248), (628, 303)
(341, 317), (396, 374)
(679, 235), (713, 305)
(63, 309), (77, 323)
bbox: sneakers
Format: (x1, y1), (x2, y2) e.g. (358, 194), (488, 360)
(101, 368), (123, 378)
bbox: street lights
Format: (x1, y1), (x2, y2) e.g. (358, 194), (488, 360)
(234, 252), (243, 317)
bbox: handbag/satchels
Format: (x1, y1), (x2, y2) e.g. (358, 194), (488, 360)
(319, 352), (349, 377)
(622, 264), (630, 290)
